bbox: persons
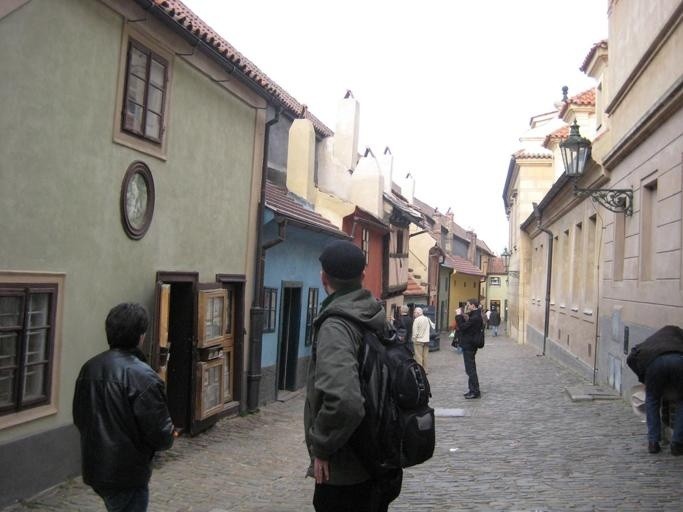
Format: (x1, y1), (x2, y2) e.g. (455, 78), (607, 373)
(455, 297), (484, 400)
(303, 240), (403, 512)
(397, 304), (413, 352)
(411, 307), (435, 375)
(627, 326), (682, 455)
(73, 303), (175, 511)
(450, 302), (501, 351)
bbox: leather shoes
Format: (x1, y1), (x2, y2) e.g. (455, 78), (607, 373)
(648, 442), (660, 453)
(463, 392), (481, 399)
(671, 442), (682, 454)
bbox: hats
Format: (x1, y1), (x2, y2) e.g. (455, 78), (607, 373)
(319, 240), (365, 278)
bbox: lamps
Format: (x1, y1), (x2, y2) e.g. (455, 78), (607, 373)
(558, 116), (634, 216)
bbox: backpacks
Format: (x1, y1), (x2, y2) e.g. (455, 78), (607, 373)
(311, 308), (435, 468)
